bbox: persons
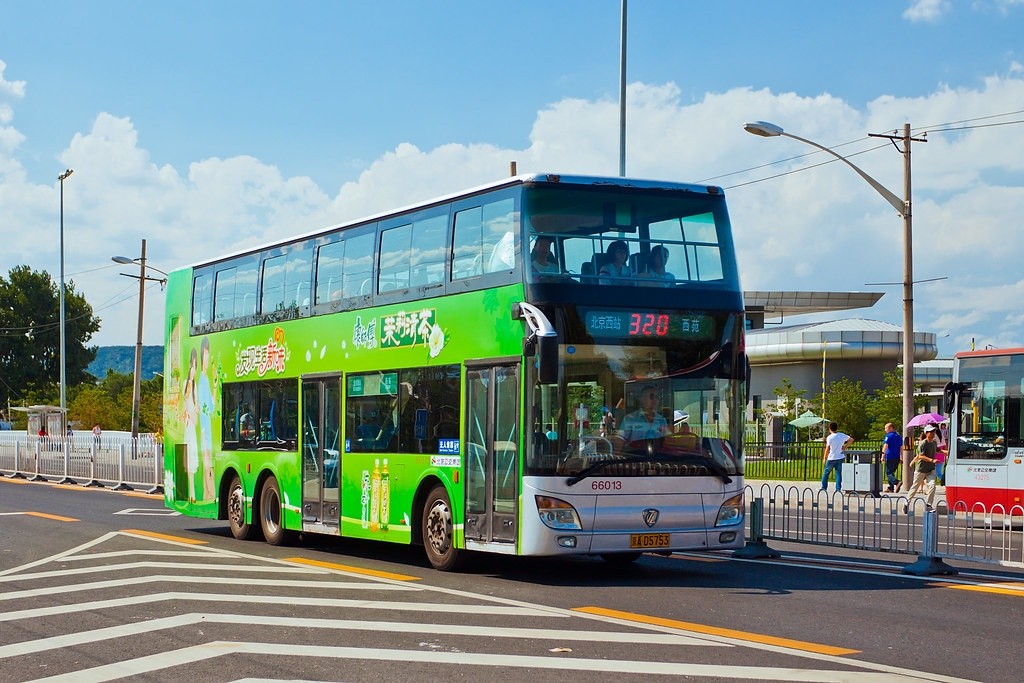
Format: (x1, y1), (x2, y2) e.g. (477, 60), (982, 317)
(67, 425), (73, 438)
(155, 427), (164, 457)
(489, 212), (520, 272)
(546, 424), (558, 441)
(92, 423), (101, 451)
(679, 423), (700, 450)
(182, 336), (219, 504)
(38, 426), (47, 443)
(530, 237), (559, 273)
(881, 423), (903, 493)
(599, 240), (632, 285)
(822, 422), (854, 491)
(356, 410), (391, 449)
(614, 385), (673, 455)
(992, 426), (1004, 444)
(239, 400), (255, 440)
(375, 382), (422, 447)
(534, 424), (548, 444)
(636, 245), (676, 287)
(903, 423), (948, 514)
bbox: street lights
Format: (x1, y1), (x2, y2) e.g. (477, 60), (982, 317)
(741, 120), (914, 492)
(56, 169), (74, 409)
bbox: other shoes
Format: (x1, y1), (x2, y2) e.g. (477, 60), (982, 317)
(896, 481), (902, 493)
(902, 501), (909, 514)
(926, 504), (936, 513)
(884, 488), (894, 492)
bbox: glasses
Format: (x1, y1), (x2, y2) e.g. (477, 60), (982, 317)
(647, 394), (659, 400)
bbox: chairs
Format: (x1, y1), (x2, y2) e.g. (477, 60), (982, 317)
(219, 242), (490, 321)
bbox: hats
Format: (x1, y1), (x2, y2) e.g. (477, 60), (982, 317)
(924, 425), (938, 435)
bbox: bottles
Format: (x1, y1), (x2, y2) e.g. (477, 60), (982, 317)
(370, 458), (390, 533)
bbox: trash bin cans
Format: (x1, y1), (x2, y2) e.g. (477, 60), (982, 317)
(841, 450), (884, 498)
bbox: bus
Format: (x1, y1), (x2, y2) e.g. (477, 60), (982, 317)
(163, 172), (747, 570)
(943, 348), (1024, 526)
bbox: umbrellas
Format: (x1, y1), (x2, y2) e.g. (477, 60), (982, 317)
(788, 410), (830, 440)
(906, 413), (947, 428)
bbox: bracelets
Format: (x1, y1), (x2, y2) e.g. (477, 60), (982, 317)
(213, 386), (217, 390)
(244, 430), (250, 437)
